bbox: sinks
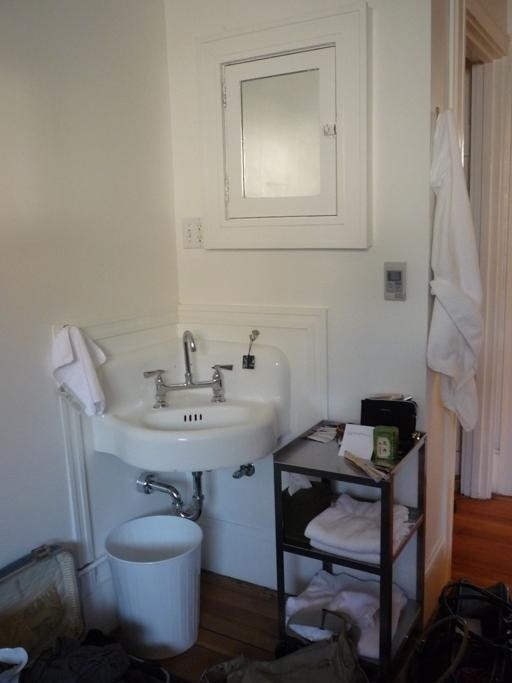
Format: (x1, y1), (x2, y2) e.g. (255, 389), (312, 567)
(90, 393), (280, 473)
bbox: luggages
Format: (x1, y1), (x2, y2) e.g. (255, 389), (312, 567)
(0, 543), (187, 683)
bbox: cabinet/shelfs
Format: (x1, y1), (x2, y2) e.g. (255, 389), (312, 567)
(270, 421), (427, 659)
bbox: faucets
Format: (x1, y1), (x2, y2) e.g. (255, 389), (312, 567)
(181, 331), (198, 384)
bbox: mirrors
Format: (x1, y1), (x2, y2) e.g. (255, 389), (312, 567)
(219, 46), (339, 218)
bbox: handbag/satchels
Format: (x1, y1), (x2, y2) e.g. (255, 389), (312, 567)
(200, 607), (368, 683)
(403, 577), (511, 682)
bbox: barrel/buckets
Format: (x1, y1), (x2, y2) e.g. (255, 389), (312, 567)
(103, 513), (203, 660)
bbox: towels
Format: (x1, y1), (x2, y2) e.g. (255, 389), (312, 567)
(304, 492), (409, 564)
(283, 569), (408, 661)
(50, 325), (108, 419)
(429, 111), (485, 433)
